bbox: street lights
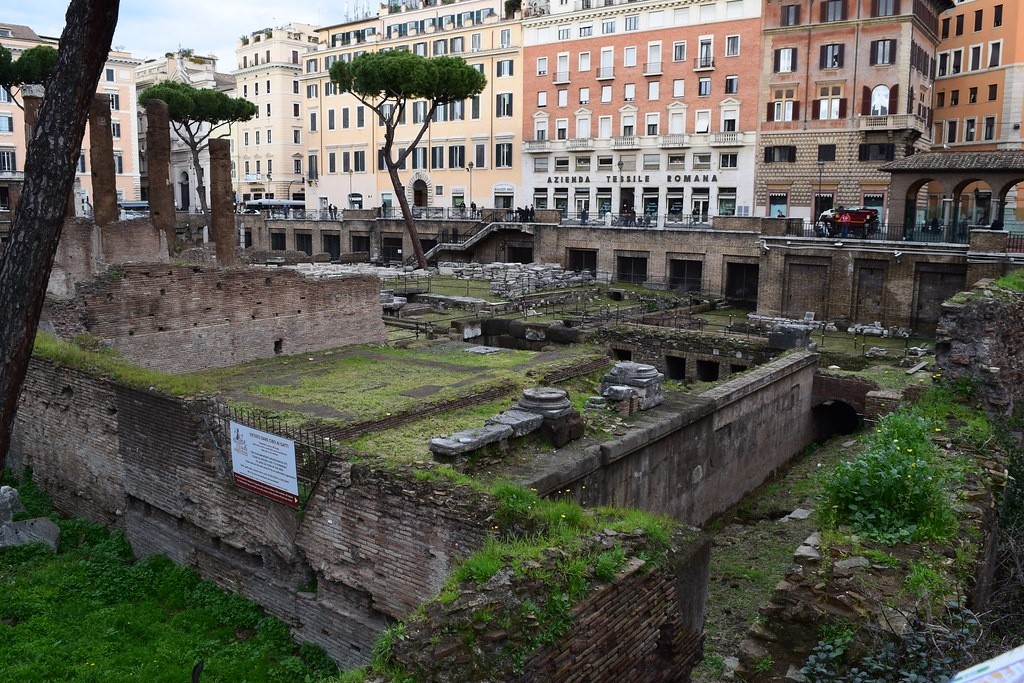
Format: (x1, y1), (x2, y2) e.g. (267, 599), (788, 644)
(814, 161), (826, 214)
(347, 168), (354, 209)
(467, 161), (475, 208)
(616, 160), (625, 214)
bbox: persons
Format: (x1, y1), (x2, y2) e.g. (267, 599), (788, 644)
(233, 200), (306, 220)
(919, 214), (1005, 243)
(382, 201), (388, 218)
(837, 211), (851, 238)
(411, 200), (536, 223)
(328, 204), (334, 220)
(333, 205), (338, 219)
(175, 205), (204, 214)
(832, 54), (839, 68)
(578, 205), (787, 229)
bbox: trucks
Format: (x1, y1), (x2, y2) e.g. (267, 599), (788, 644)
(815, 205), (880, 240)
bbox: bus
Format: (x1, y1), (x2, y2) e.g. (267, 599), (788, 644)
(117, 199), (178, 216)
(245, 198), (305, 216)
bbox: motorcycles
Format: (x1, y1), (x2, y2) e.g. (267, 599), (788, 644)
(921, 220), (941, 234)
